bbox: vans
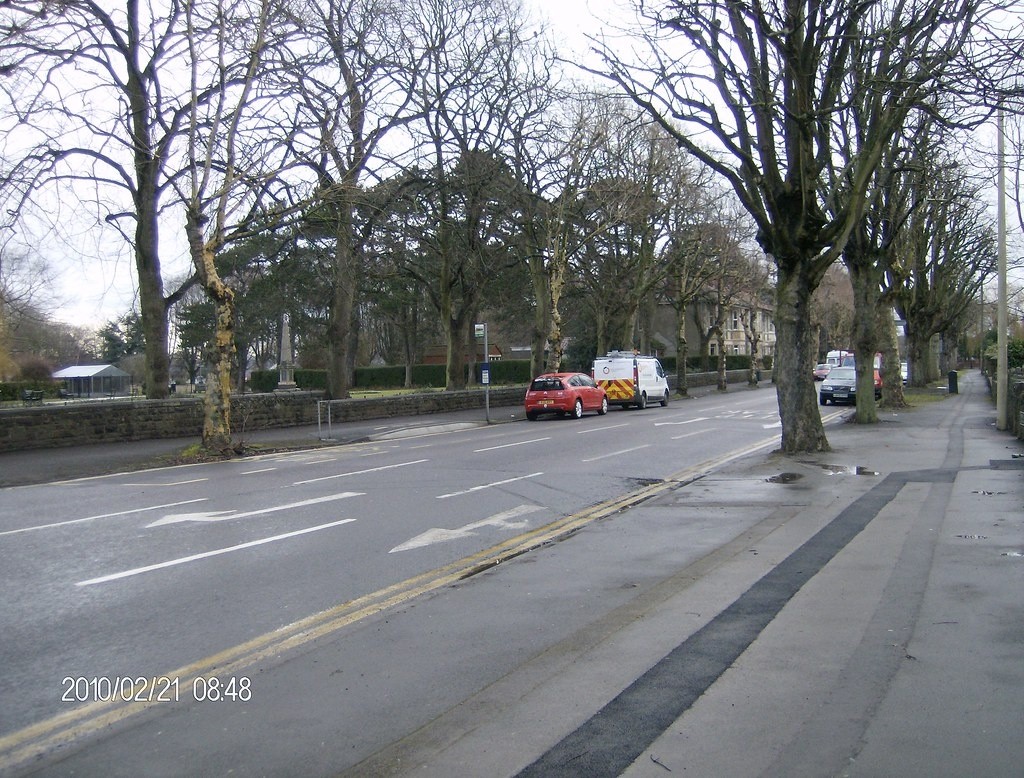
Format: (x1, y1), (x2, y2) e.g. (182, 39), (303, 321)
(591, 349), (669, 409)
(827, 349), (849, 368)
(843, 352), (883, 401)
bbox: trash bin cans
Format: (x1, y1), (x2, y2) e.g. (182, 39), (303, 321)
(947, 369), (960, 394)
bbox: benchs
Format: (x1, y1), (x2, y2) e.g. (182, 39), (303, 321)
(23, 390), (43, 407)
(105, 390), (116, 399)
(59, 389), (74, 400)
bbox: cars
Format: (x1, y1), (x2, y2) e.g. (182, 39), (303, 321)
(819, 366), (857, 406)
(812, 364), (838, 382)
(900, 363), (907, 384)
(524, 371), (609, 420)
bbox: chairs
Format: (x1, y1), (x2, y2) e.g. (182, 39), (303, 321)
(541, 383), (559, 388)
